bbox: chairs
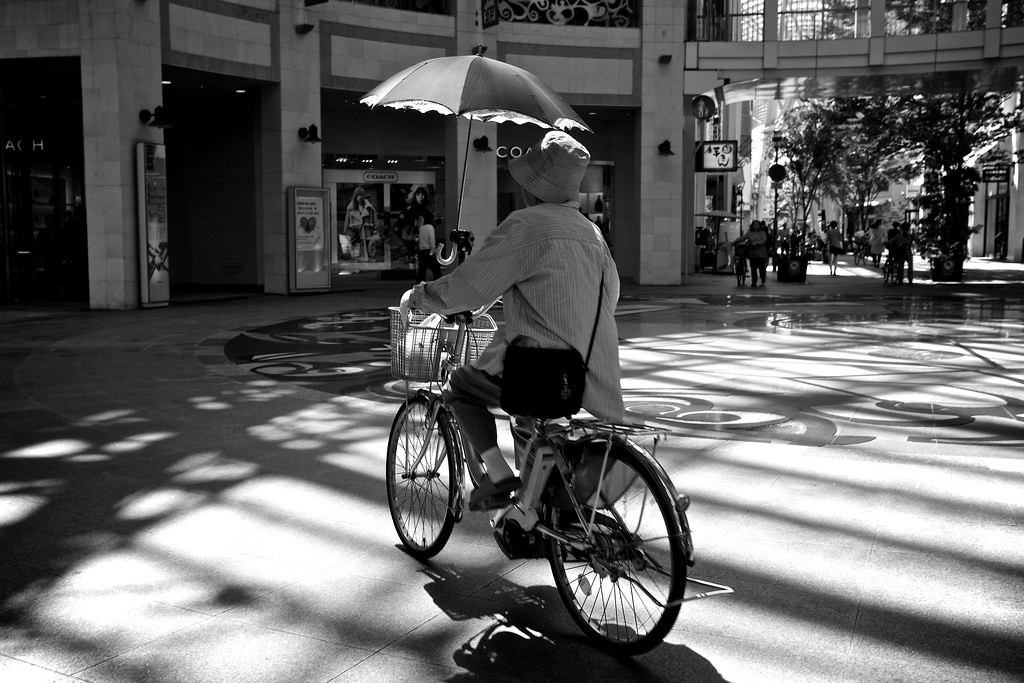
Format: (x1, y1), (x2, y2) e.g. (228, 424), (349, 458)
(695, 246), (719, 273)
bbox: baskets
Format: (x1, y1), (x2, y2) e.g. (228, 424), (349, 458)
(386, 304), (497, 382)
(734, 244), (749, 258)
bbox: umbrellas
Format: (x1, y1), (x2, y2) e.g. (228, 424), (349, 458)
(352, 45), (595, 266)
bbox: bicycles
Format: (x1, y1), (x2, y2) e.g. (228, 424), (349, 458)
(881, 247), (906, 286)
(732, 243), (751, 287)
(854, 237), (871, 266)
(385, 295), (696, 657)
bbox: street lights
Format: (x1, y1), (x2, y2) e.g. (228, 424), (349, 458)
(773, 130), (783, 271)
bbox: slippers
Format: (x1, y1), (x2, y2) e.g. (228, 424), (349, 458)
(469, 474), (523, 511)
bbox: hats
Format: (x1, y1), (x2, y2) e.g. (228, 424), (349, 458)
(507, 131), (591, 205)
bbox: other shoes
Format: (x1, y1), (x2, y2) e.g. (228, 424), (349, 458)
(751, 283), (756, 287)
(897, 281), (903, 285)
(761, 283), (765, 286)
(907, 282), (912, 286)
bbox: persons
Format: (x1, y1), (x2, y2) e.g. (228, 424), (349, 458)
(826, 221), (843, 275)
(415, 215), (442, 284)
(342, 185), (379, 263)
(883, 221), (921, 285)
(777, 223), (790, 256)
(866, 219), (887, 268)
(400, 130), (625, 512)
(735, 220), (768, 288)
(760, 221), (774, 269)
(393, 187), (434, 270)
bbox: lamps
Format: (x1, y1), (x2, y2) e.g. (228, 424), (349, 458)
(657, 140), (676, 156)
(138, 105), (172, 130)
(474, 136), (493, 153)
(297, 124), (322, 144)
(658, 55), (672, 64)
(296, 24), (315, 34)
(473, 45), (488, 54)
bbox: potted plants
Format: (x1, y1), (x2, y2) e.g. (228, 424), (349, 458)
(765, 85), (1024, 282)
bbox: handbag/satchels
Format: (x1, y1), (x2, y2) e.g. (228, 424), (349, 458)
(500, 343), (586, 419)
